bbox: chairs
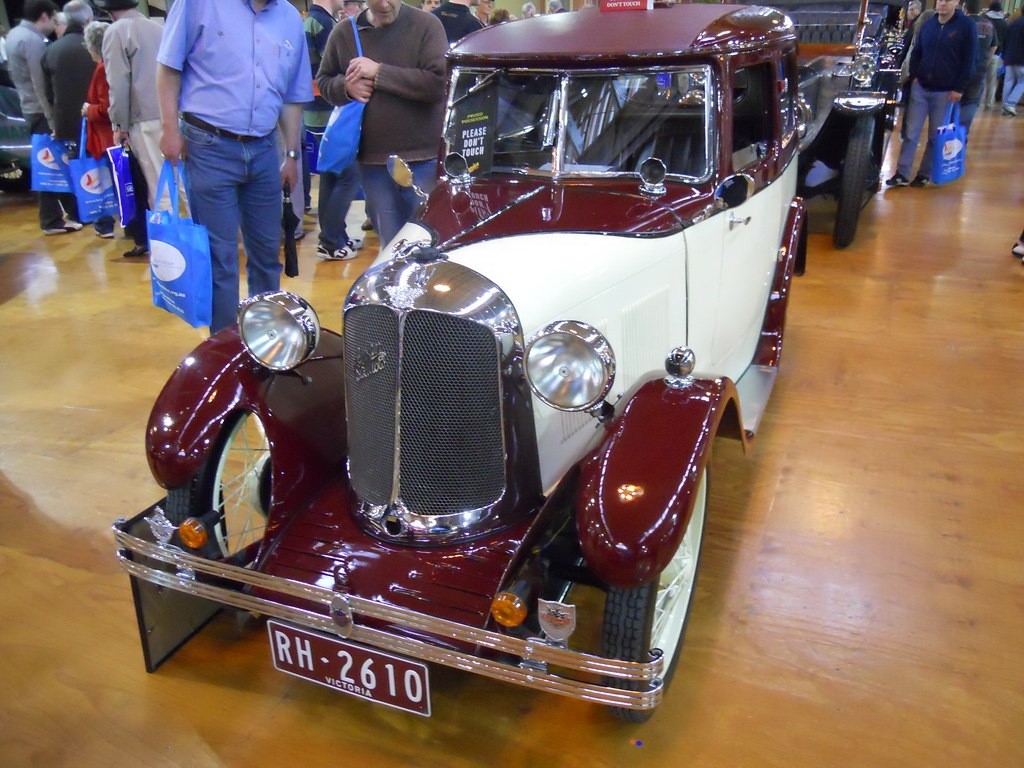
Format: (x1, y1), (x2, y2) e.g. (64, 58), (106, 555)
(613, 109), (706, 177)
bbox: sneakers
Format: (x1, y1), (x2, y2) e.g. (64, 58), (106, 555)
(886, 174), (909, 185)
(317, 244), (357, 260)
(911, 173), (929, 187)
(346, 239), (363, 250)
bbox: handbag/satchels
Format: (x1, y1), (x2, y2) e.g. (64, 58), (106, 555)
(107, 144), (137, 228)
(933, 101), (966, 187)
(146, 158), (212, 328)
(316, 101), (366, 173)
(32, 133), (73, 193)
(69, 118), (118, 223)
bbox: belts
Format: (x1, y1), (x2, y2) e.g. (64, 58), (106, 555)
(182, 112), (260, 141)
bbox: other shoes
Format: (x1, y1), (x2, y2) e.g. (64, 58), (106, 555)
(43, 223), (83, 234)
(1003, 100), (1019, 116)
(123, 244), (147, 257)
(96, 231), (114, 237)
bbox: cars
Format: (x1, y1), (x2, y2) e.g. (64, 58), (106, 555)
(677, 0), (912, 248)
(111, 0), (815, 721)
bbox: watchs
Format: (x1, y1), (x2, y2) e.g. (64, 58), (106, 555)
(283, 150), (300, 160)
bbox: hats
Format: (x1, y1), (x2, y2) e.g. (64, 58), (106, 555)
(93, 0), (139, 8)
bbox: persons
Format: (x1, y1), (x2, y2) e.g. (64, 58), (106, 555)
(315, 0), (449, 252)
(905, 0), (1024, 147)
(0, 0), (571, 258)
(156, 0), (314, 337)
(886, 0), (978, 188)
